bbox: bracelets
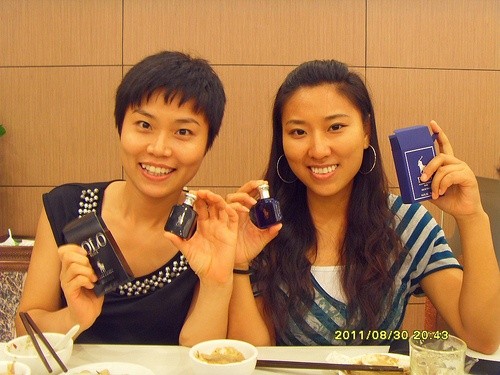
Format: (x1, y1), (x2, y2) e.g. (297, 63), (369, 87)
(233, 268), (250, 275)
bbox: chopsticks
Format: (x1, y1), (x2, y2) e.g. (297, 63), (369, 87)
(18, 311), (68, 373)
(256, 360), (403, 372)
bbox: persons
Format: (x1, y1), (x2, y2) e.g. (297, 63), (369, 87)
(226, 58), (500, 356)
(14, 49), (239, 347)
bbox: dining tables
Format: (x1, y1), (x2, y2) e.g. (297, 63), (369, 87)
(0, 343), (500, 375)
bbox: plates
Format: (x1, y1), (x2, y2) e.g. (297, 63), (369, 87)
(339, 353), (410, 375)
(61, 361), (155, 375)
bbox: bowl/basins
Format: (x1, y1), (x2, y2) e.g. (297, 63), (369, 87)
(0, 361), (31, 375)
(5, 333), (73, 375)
(190, 340), (258, 375)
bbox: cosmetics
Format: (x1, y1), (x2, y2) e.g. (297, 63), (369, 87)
(252, 184), (283, 229)
(163, 186), (201, 240)
(388, 125), (445, 205)
(63, 211), (135, 299)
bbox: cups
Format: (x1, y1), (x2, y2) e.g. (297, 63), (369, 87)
(409, 332), (478, 375)
